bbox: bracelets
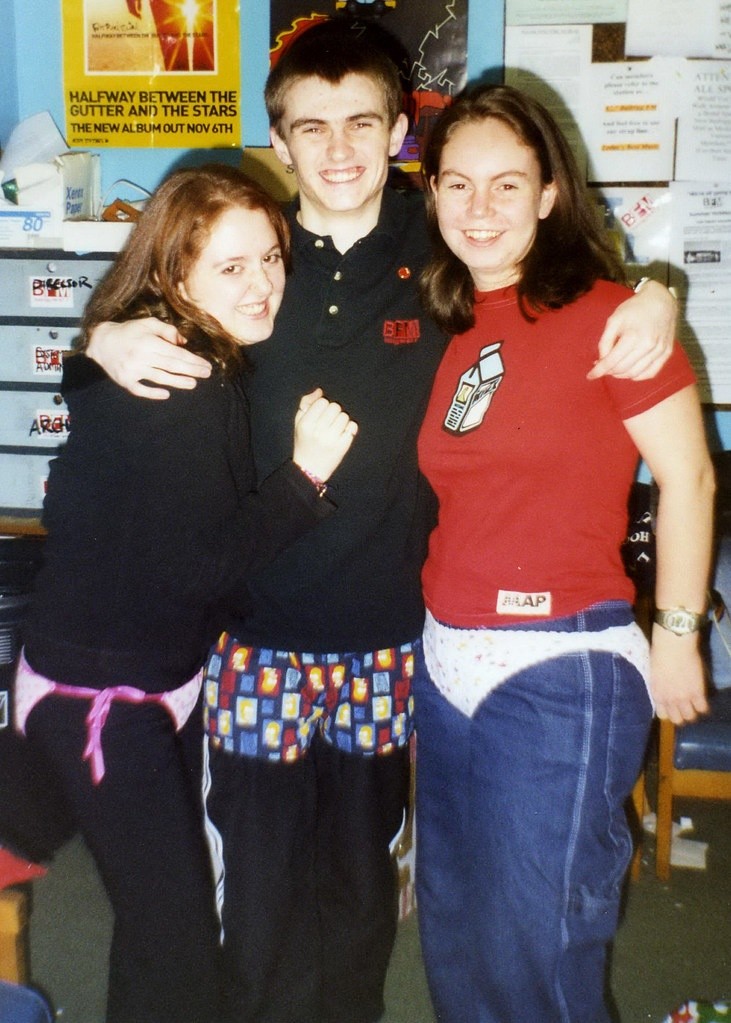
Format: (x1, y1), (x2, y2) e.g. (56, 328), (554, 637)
(299, 465), (326, 499)
(633, 275), (654, 294)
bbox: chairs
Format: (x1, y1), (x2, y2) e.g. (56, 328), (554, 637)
(612, 591), (730, 884)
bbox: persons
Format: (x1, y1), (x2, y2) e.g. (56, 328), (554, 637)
(16, 166), (359, 1021)
(415, 81), (716, 1021)
(79, 14), (679, 1023)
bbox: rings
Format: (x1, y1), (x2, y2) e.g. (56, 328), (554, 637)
(297, 405), (306, 414)
(342, 430), (356, 439)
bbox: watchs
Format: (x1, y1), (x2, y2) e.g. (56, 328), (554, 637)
(653, 603), (705, 637)
